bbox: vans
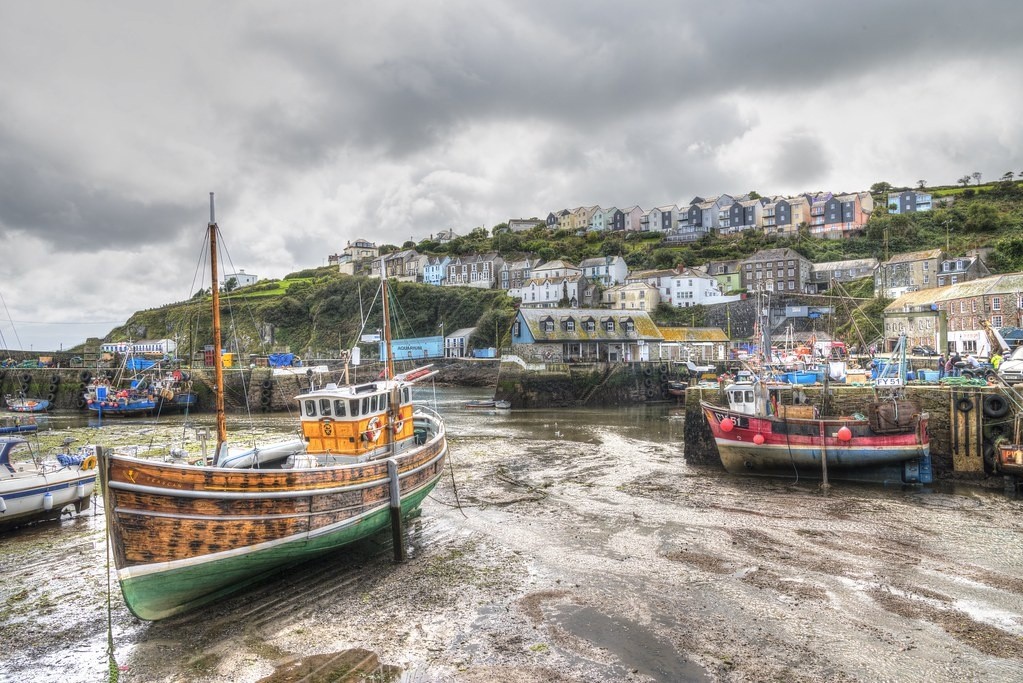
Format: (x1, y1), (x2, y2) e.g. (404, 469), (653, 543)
(998, 345), (1023, 386)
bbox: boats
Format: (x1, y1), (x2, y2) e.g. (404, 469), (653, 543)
(4, 393), (49, 411)
(95, 191), (446, 622)
(697, 274), (935, 486)
(0, 415), (95, 533)
(152, 333), (198, 410)
(495, 399), (511, 408)
(665, 360), (717, 397)
(464, 401), (495, 407)
(82, 336), (155, 416)
(983, 369), (1023, 482)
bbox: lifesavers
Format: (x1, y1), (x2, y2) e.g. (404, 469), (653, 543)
(83, 454), (96, 470)
(983, 395), (1010, 416)
(959, 368), (995, 381)
(368, 415), (383, 442)
(0, 371), (5, 392)
(76, 370), (90, 408)
(261, 380), (271, 406)
(660, 365), (669, 390)
(18, 374), (32, 399)
(984, 446), (997, 466)
(394, 411), (404, 433)
(956, 396), (973, 412)
(985, 419), (1011, 442)
(47, 374), (59, 402)
(645, 368), (654, 397)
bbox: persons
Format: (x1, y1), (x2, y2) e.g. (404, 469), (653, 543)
(946, 358), (953, 372)
(815, 406), (819, 418)
(870, 345), (876, 357)
(938, 354), (945, 379)
(964, 354), (980, 367)
(951, 352), (962, 376)
(988, 349), (1003, 371)
(1002, 348), (1010, 361)
